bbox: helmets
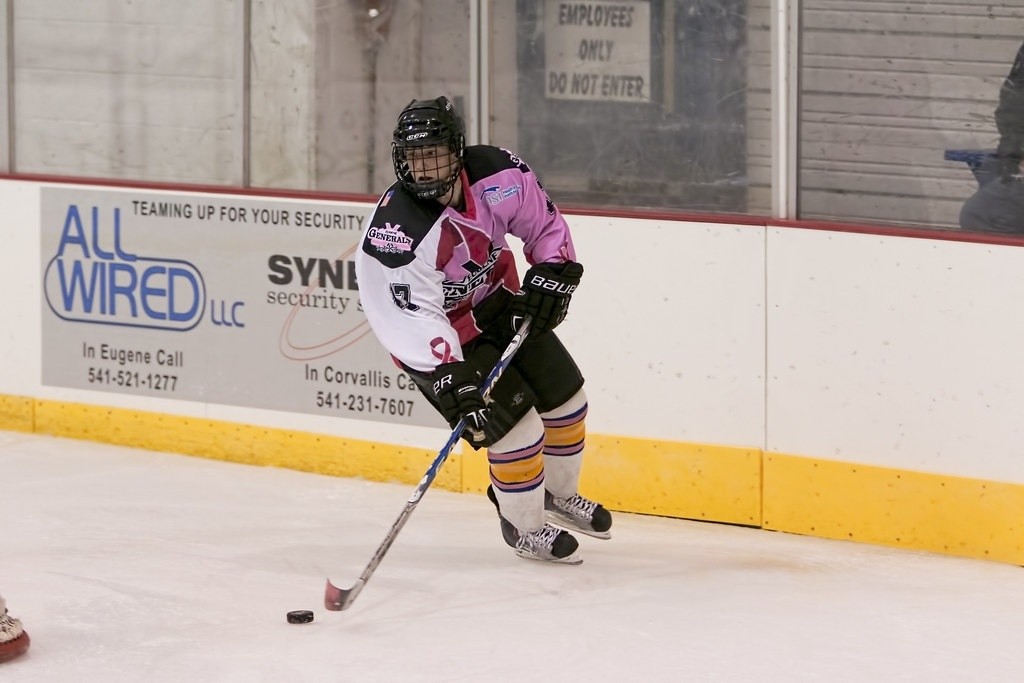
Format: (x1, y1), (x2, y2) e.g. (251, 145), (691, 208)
(393, 96), (466, 166)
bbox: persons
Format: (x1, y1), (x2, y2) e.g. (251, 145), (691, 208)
(354, 95), (611, 565)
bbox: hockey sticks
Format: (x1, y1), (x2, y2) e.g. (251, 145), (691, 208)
(323, 313), (534, 610)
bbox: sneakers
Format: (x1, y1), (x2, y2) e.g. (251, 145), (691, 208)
(545, 488), (613, 540)
(487, 484), (584, 565)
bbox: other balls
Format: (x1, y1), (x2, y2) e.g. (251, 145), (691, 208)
(286, 608), (316, 626)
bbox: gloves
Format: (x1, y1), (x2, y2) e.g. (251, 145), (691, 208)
(433, 361), (489, 431)
(507, 260), (583, 339)
